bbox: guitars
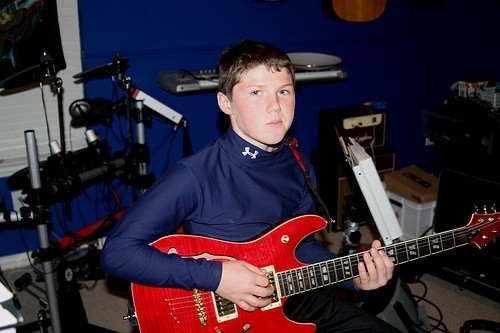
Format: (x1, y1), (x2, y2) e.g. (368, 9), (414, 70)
(130, 204), (500, 333)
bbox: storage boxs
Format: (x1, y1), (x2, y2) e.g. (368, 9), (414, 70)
(381, 165), (440, 244)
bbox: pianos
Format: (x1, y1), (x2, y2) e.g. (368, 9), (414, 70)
(158, 54), (347, 95)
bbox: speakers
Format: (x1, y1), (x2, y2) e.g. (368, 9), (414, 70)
(314, 148), (395, 232)
(425, 169), (500, 302)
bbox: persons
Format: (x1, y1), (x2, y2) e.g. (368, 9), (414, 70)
(100, 38), (404, 333)
